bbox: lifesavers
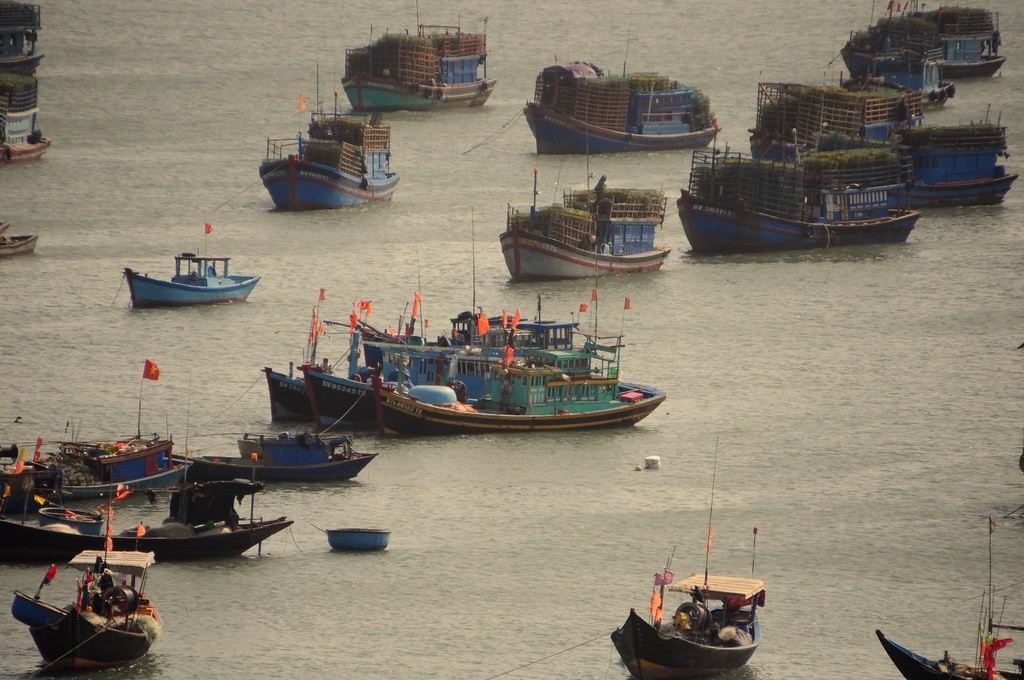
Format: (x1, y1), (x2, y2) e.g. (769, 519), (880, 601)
(411, 82), (419, 94)
(946, 84), (955, 98)
(805, 224), (816, 239)
(425, 86), (433, 100)
(353, 374), (362, 382)
(437, 88), (448, 103)
(361, 178), (368, 190)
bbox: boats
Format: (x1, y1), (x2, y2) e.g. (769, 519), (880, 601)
(259, 61), (399, 210)
(524, 51), (721, 156)
(122, 224), (261, 309)
(10, 464), (162, 670)
(296, 206), (666, 434)
(676, 121), (922, 253)
(34, 358), (192, 498)
(0, 112), (52, 164)
(843, 53), (956, 110)
(340, 23), (496, 113)
(841, 0), (1007, 78)
(747, 82), (1020, 210)
(610, 435), (765, 680)
(499, 170), (671, 283)
(260, 250), (447, 424)
(0, 222), (39, 258)
(0, 443), (73, 513)
(171, 431), (379, 482)
(0, 516), (294, 560)
(876, 514), (1023, 680)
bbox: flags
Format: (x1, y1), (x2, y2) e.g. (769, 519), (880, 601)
(205, 224), (211, 235)
(478, 308), (520, 364)
(46, 564), (58, 581)
(107, 483), (132, 552)
(654, 570), (674, 586)
(349, 300), (372, 334)
(652, 591), (663, 624)
(142, 360), (160, 380)
(137, 523), (146, 538)
(980, 633), (1014, 680)
(625, 296), (631, 309)
(299, 94), (305, 109)
(308, 289), (326, 343)
(3, 436), (46, 505)
(405, 293), (428, 337)
(708, 526), (713, 557)
(990, 518), (998, 533)
(592, 289), (597, 301)
(390, 327), (396, 337)
(580, 304), (588, 312)
